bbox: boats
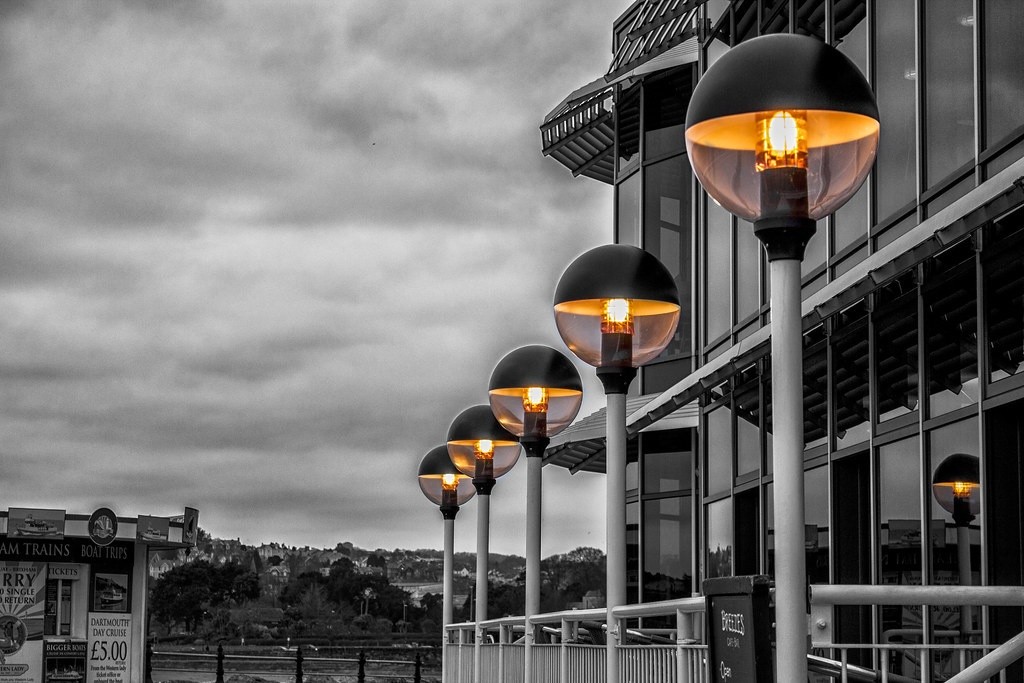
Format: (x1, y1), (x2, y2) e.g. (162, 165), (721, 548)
(281, 646), (298, 652)
(100, 590), (125, 606)
(139, 520), (168, 542)
(16, 512), (59, 537)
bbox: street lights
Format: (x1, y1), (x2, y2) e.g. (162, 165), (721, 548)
(416, 442), (476, 683)
(403, 605), (408, 622)
(487, 343), (582, 683)
(552, 242), (680, 683)
(445, 402), (525, 683)
(933, 448), (981, 659)
(470, 586), (474, 622)
(683, 33), (881, 683)
